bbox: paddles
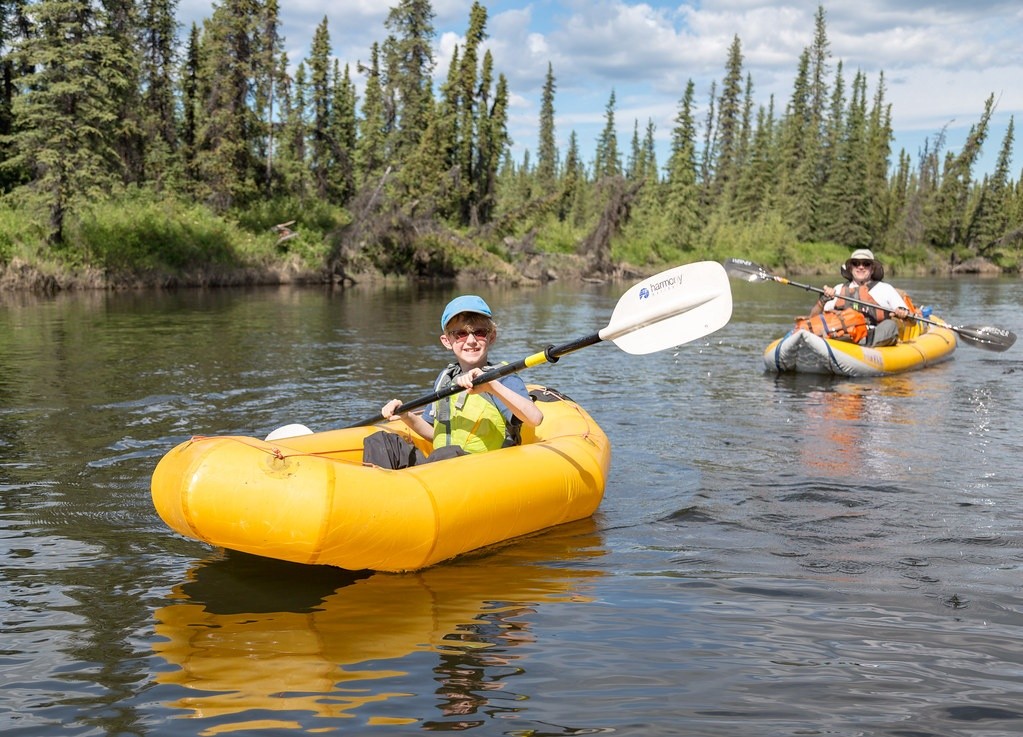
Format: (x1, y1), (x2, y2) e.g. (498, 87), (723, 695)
(269, 261), (733, 441)
(723, 254), (1015, 350)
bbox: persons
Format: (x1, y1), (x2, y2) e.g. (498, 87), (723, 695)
(809, 248), (917, 348)
(362, 295), (543, 471)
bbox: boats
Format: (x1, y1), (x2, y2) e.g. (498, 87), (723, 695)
(151, 383), (612, 572)
(764, 310), (956, 377)
(152, 510), (617, 737)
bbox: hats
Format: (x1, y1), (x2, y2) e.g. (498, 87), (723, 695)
(441, 295), (492, 334)
(841, 248), (885, 281)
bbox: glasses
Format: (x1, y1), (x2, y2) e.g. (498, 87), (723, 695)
(448, 328), (490, 339)
(851, 260), (871, 267)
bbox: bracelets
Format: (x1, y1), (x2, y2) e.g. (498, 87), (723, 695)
(817, 296), (827, 307)
(900, 307), (909, 311)
